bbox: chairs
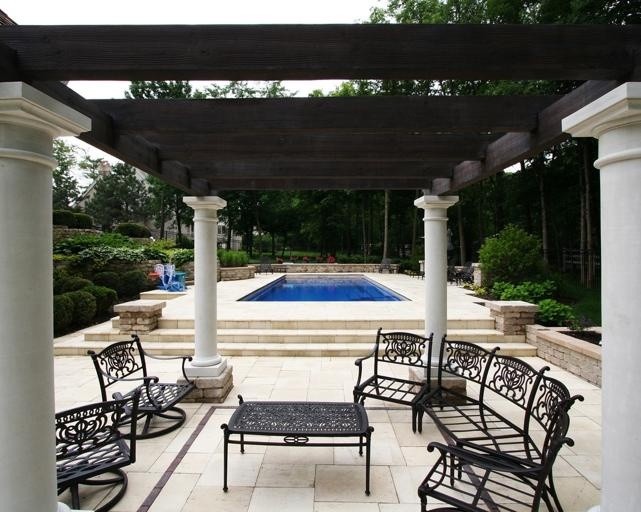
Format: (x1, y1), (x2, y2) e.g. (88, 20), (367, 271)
(375, 258), (474, 286)
(418, 398), (576, 510)
(55, 387), (142, 511)
(353, 327), (434, 432)
(88, 332), (199, 438)
(259, 256), (273, 274)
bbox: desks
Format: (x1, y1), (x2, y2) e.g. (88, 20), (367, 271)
(275, 268), (288, 272)
(221, 394), (374, 496)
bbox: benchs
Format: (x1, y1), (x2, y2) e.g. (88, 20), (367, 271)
(419, 335), (584, 479)
